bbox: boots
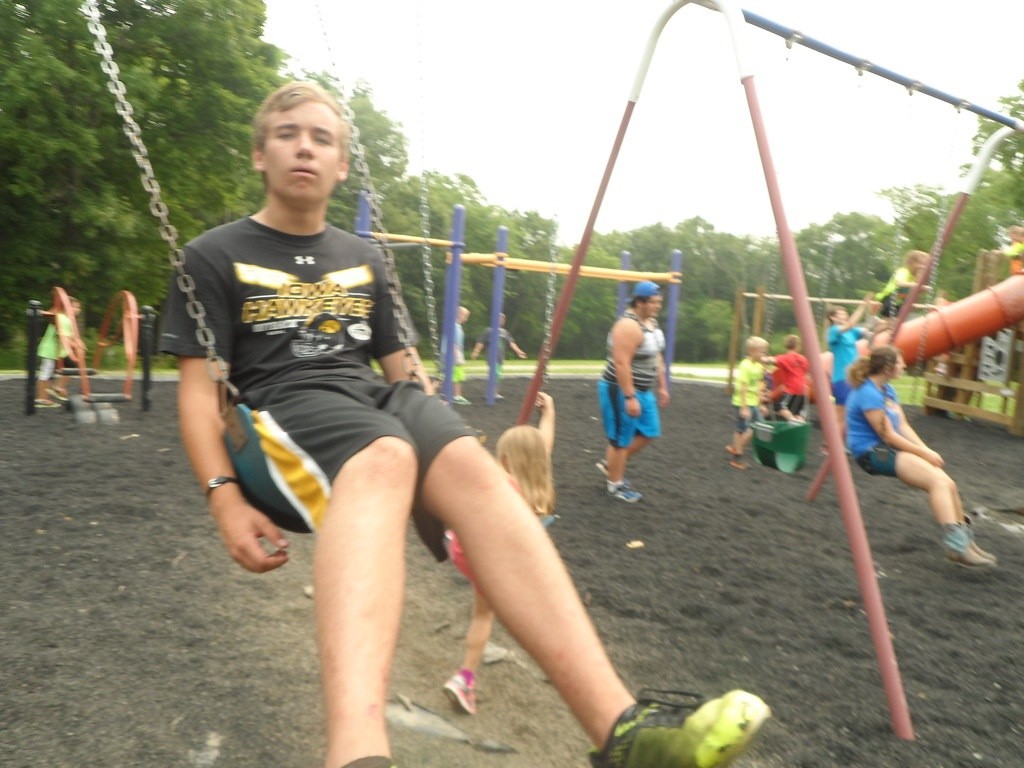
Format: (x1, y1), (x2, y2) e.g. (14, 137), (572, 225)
(959, 524), (997, 564)
(942, 522), (996, 572)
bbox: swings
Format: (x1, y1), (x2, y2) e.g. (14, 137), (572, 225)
(856, 90), (963, 475)
(413, 0), (572, 535)
(80, 0), (427, 533)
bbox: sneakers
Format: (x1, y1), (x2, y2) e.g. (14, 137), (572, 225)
(589, 688), (770, 768)
(442, 671), (478, 715)
(596, 458), (608, 473)
(604, 479), (642, 502)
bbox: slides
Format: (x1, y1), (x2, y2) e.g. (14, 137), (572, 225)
(808, 268), (1024, 372)
(772, 272), (1024, 404)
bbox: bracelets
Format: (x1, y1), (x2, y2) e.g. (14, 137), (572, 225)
(206, 476), (239, 500)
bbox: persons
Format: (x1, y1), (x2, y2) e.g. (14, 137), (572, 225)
(471, 313), (527, 399)
(34, 297), (82, 407)
(993, 225), (1024, 275)
(450, 306), (470, 404)
(595, 282), (670, 502)
(820, 292), (873, 458)
(163, 85), (770, 768)
(848, 346), (996, 569)
(443, 390), (555, 715)
(877, 250), (940, 330)
(725, 335), (809, 469)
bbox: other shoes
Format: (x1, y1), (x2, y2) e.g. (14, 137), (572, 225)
(730, 459), (753, 470)
(44, 385), (68, 401)
(726, 445), (743, 458)
(35, 399), (60, 409)
(451, 396), (470, 405)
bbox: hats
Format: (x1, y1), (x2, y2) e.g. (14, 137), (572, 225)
(623, 281), (659, 304)
